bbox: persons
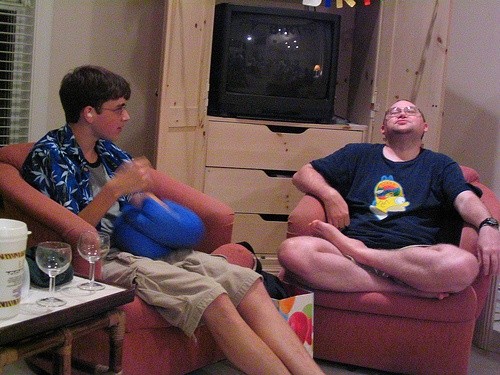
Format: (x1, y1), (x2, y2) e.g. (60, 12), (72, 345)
(21, 64), (323, 375)
(277, 98), (499, 300)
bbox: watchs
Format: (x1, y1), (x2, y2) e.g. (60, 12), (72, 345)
(478, 217), (499, 232)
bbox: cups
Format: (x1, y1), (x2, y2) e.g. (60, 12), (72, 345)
(1, 218), (28, 318)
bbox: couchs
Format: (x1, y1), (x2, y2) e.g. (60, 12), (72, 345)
(0, 143), (257, 375)
(277, 167), (500, 375)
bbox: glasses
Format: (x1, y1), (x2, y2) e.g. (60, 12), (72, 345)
(385, 106), (419, 116)
(88, 104), (128, 114)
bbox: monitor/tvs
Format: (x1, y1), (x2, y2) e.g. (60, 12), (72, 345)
(207, 2), (341, 124)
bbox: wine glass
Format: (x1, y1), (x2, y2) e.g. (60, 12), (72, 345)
(77, 231), (110, 290)
(36, 241), (72, 306)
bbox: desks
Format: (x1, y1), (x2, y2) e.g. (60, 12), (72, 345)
(0, 271), (136, 375)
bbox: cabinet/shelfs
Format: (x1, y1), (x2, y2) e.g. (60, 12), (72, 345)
(156, 117), (368, 277)
